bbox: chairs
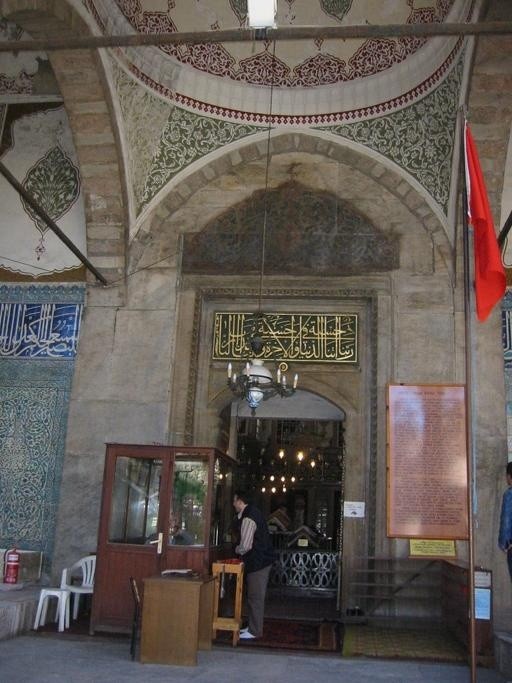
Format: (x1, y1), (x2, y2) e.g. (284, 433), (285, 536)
(57, 554), (99, 625)
(128, 573), (144, 661)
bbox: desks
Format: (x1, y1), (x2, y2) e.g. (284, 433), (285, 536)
(142, 570), (218, 669)
(210, 558), (244, 648)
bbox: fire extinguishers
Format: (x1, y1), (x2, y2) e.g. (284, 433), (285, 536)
(3, 547), (20, 584)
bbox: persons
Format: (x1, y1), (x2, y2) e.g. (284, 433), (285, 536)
(233, 491), (273, 639)
(498, 461), (512, 577)
(145, 516), (193, 545)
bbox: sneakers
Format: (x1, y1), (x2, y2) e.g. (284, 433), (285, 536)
(239, 625), (249, 633)
(239, 631), (256, 639)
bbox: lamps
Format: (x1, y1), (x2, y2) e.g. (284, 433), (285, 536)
(222, 31), (304, 419)
(243, 0), (281, 29)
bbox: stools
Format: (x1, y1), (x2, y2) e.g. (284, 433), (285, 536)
(31, 586), (73, 633)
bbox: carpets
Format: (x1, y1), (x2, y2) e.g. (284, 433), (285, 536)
(337, 618), (469, 665)
(32, 612), (341, 660)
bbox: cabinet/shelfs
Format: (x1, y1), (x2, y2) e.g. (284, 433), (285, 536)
(349, 553), (442, 605)
(441, 560), (494, 655)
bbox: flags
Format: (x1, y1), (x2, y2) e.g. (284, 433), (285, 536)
(464, 122), (506, 321)
